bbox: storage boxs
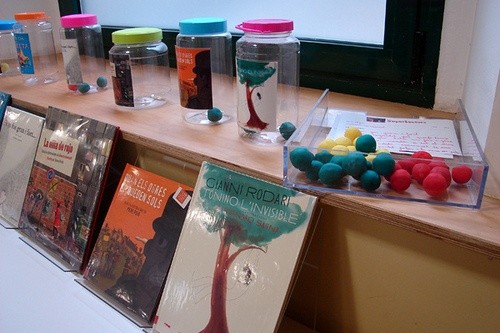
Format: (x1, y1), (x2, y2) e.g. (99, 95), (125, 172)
(282, 88), (491, 210)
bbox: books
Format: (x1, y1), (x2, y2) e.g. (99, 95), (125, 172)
(149, 160), (323, 333)
(16, 105), (119, 276)
(83, 161), (195, 321)
(0, 90), (12, 128)
(0, 106), (47, 229)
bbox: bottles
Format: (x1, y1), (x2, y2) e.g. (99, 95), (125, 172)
(12, 11), (59, 86)
(175, 18), (233, 126)
(0, 19), (20, 76)
(108, 28), (172, 111)
(234, 18), (301, 148)
(59, 14), (111, 96)
